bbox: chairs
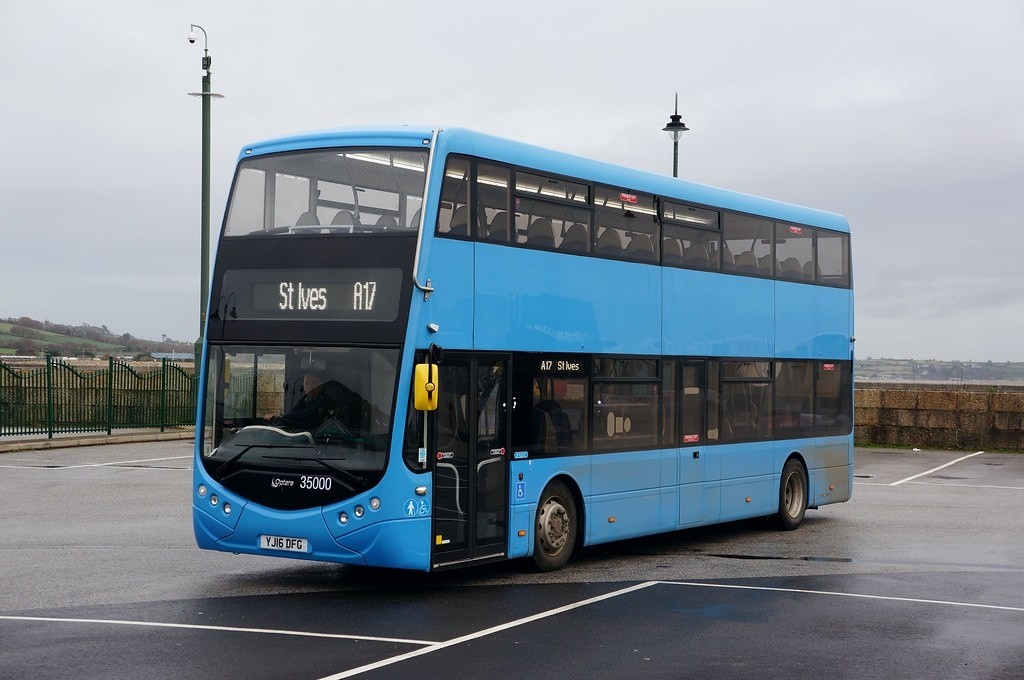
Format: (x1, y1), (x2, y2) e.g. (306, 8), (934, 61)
(323, 379), (351, 428)
(293, 207), (822, 285)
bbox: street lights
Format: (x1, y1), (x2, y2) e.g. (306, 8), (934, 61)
(663, 91), (689, 179)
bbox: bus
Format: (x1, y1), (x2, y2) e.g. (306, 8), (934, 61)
(191, 126), (855, 575)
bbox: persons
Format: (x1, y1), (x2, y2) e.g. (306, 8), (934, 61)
(265, 369), (339, 434)
(572, 366), (853, 453)
